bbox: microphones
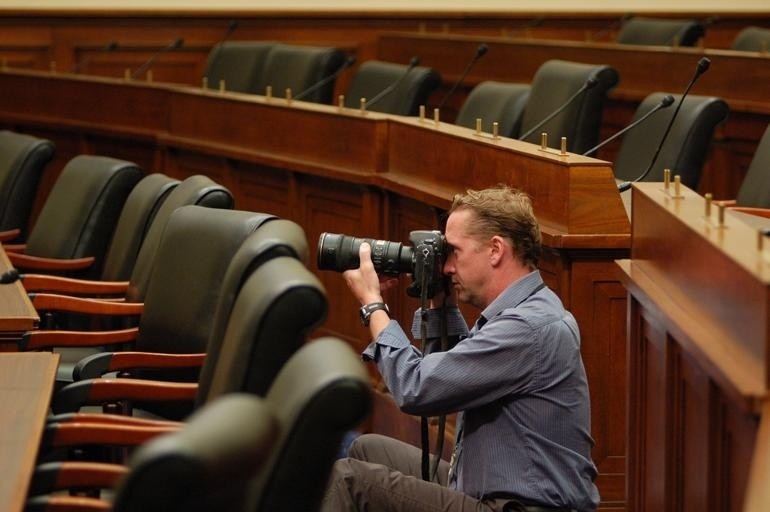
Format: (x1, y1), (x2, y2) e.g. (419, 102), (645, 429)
(432, 41), (489, 120)
(360, 55), (421, 109)
(583, 94), (675, 154)
(0, 269), (20, 285)
(210, 19), (240, 85)
(520, 73), (601, 144)
(128, 35), (183, 78)
(64, 40), (116, 76)
(288, 55), (357, 104)
(618, 57), (713, 190)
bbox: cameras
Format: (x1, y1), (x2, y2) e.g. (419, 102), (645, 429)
(314, 228), (449, 299)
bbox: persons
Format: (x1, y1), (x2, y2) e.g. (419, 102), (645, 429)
(322, 186), (602, 510)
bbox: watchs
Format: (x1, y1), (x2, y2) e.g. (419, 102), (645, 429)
(359, 303), (391, 325)
